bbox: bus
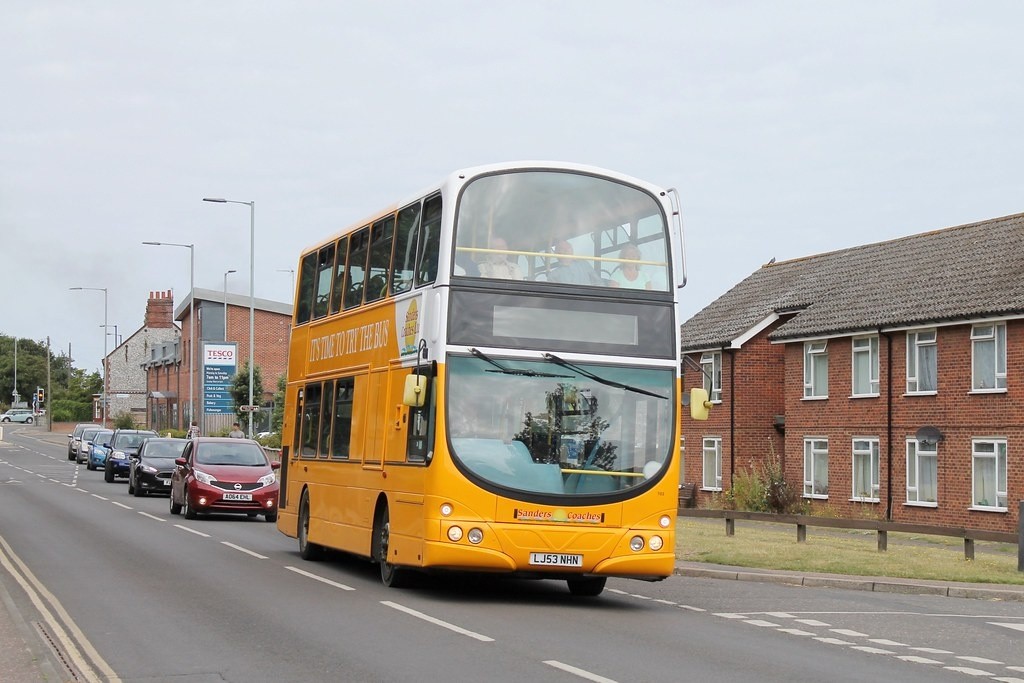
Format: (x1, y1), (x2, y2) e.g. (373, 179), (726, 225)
(276, 162), (715, 600)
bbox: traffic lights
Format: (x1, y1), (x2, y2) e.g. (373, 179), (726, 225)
(37, 389), (44, 402)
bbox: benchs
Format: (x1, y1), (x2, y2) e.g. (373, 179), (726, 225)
(678, 481), (698, 508)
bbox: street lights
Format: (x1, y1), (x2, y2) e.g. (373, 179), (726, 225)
(107, 333), (122, 345)
(224, 270), (236, 341)
(100, 325), (117, 348)
(142, 241), (194, 432)
(202, 198), (255, 439)
(69, 288), (108, 426)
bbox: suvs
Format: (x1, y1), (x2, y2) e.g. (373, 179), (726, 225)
(67, 423), (105, 461)
(103, 427), (160, 483)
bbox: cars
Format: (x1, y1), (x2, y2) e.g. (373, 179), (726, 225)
(86, 432), (114, 470)
(75, 428), (113, 464)
(128, 437), (192, 497)
(169, 436), (281, 522)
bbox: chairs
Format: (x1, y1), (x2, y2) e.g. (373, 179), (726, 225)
(294, 263), (636, 468)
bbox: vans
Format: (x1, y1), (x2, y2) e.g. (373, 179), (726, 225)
(0, 408), (34, 424)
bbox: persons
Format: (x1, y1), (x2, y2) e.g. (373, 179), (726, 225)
(477, 237), (524, 279)
(548, 241), (605, 287)
(186, 421), (201, 439)
(229, 423), (245, 438)
(611, 243), (653, 288)
(427, 237), (481, 282)
